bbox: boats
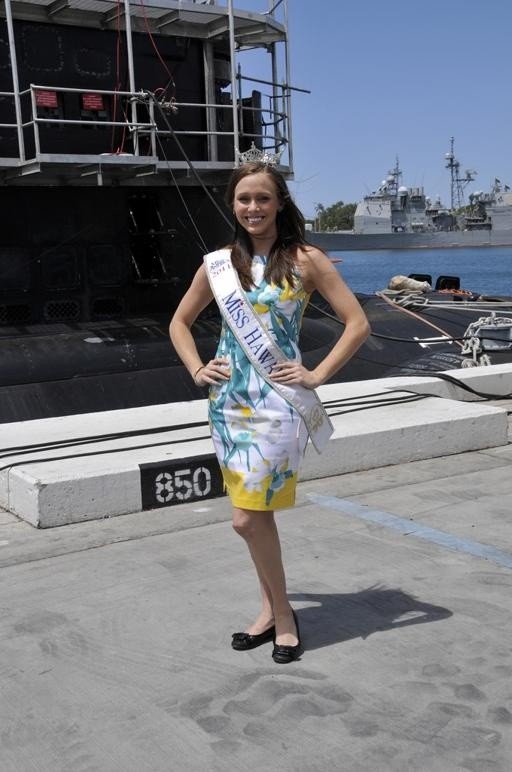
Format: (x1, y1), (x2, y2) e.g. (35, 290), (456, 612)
(302, 138), (511, 252)
(1, 0), (512, 428)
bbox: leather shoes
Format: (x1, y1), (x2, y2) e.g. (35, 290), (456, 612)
(231, 625), (275, 650)
(272, 609), (301, 663)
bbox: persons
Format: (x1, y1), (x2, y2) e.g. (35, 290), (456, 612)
(166, 161), (371, 664)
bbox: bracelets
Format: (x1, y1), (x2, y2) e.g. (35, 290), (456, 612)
(194, 366), (205, 378)
